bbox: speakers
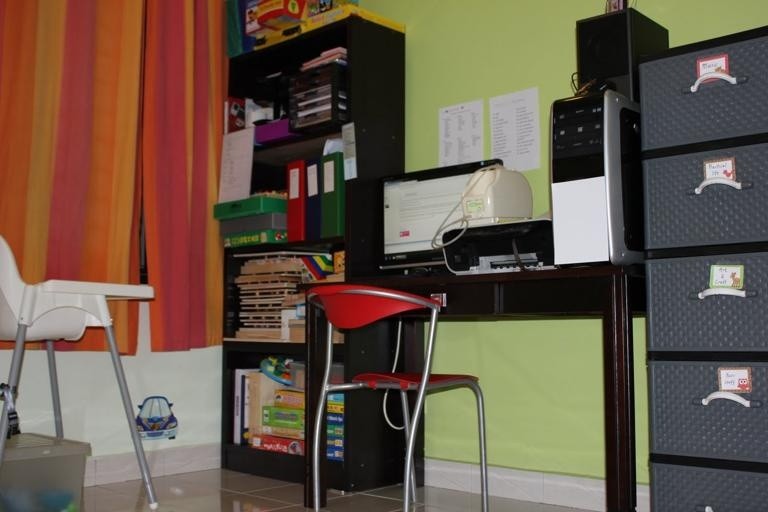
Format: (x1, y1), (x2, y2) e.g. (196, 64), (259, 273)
(576, 9), (669, 102)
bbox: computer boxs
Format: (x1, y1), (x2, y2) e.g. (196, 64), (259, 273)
(548, 90), (646, 266)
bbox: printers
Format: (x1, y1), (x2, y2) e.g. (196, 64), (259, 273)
(439, 219), (559, 277)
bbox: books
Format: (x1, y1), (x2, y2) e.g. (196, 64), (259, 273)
(299, 46), (348, 73)
(222, 95), (246, 135)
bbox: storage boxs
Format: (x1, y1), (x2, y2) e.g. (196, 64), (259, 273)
(0, 431), (93, 512)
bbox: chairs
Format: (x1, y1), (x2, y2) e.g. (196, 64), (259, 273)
(0, 233), (161, 512)
(303, 283), (490, 512)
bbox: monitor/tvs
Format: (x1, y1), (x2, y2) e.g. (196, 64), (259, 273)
(377, 159), (503, 271)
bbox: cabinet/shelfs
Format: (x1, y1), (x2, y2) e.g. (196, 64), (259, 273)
(220, 14), (407, 492)
(633, 24), (768, 512)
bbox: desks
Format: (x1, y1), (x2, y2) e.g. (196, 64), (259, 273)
(359, 264), (647, 512)
